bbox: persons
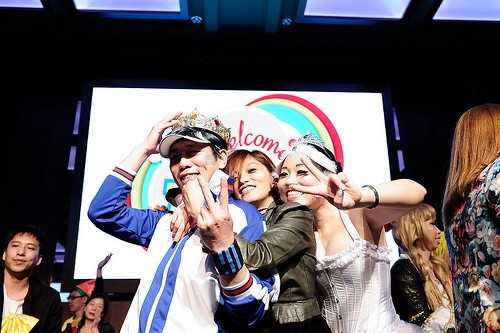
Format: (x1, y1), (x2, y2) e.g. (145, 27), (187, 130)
(61, 109), (454, 333)
(0, 226), (62, 333)
(441, 103), (500, 333)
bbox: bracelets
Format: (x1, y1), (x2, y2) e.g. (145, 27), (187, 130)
(363, 185), (379, 210)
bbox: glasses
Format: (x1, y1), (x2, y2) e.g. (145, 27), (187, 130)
(67, 294), (86, 300)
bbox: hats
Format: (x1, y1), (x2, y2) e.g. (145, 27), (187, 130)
(159, 113), (231, 157)
(76, 279), (95, 298)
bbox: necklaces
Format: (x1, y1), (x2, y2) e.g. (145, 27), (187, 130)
(259, 208), (268, 212)
